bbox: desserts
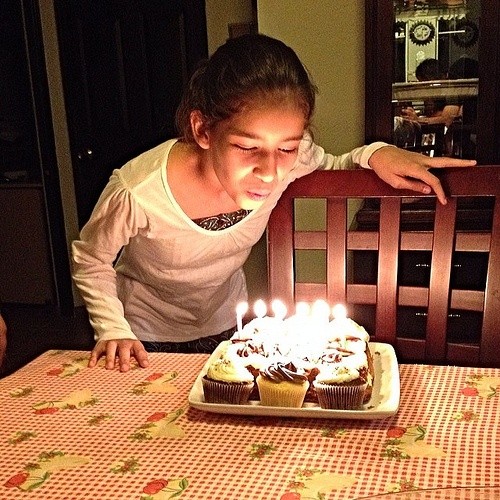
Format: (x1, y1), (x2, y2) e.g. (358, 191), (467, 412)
(256, 361), (310, 410)
(200, 359), (255, 405)
(313, 365), (368, 410)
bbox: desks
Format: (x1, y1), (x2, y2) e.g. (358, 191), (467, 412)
(0, 349), (500, 500)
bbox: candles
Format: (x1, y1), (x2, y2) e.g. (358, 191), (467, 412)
(234, 298), (348, 351)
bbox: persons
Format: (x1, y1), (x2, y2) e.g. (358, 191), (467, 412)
(67, 28), (479, 374)
(0, 311), (11, 381)
(394, 58), (479, 161)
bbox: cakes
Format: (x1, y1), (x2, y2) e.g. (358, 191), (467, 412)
(219, 316), (375, 404)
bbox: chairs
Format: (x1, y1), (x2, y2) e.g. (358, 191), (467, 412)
(261, 164), (500, 369)
(396, 124), (446, 158)
